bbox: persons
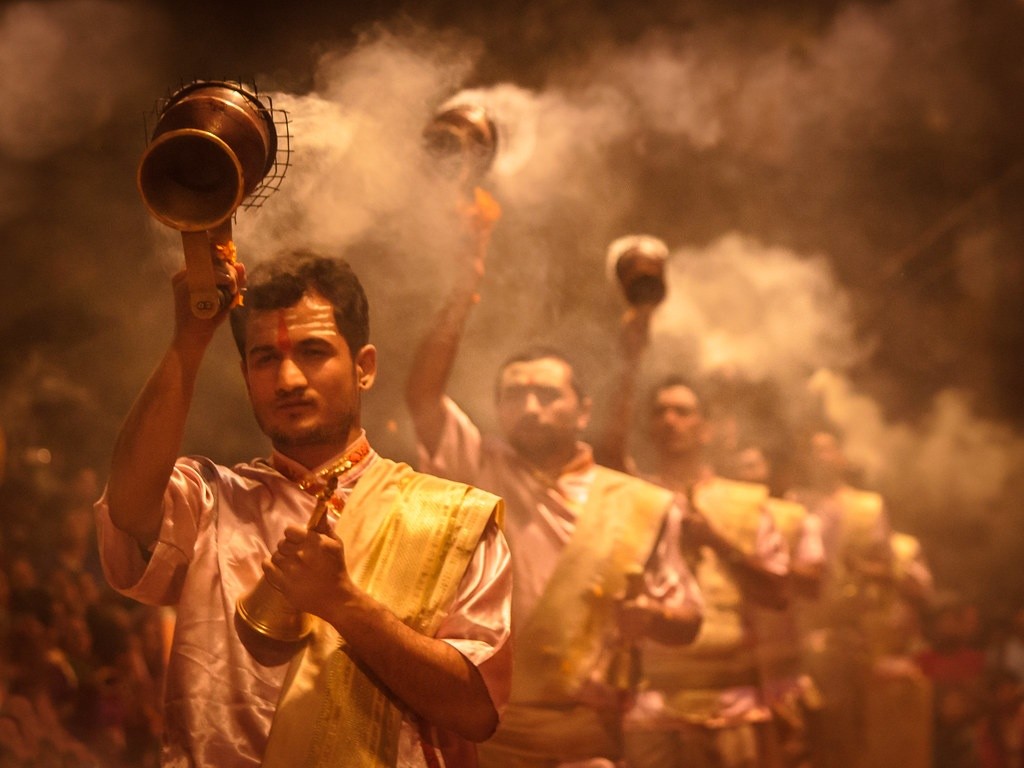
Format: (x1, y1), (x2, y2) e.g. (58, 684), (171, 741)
(94, 183), (935, 768)
